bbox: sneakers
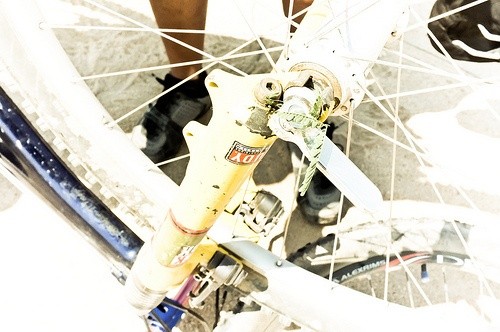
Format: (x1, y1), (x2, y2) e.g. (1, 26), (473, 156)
(126, 66), (214, 165)
(292, 120), (348, 226)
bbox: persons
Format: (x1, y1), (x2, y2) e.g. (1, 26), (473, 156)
(135, 0), (344, 225)
